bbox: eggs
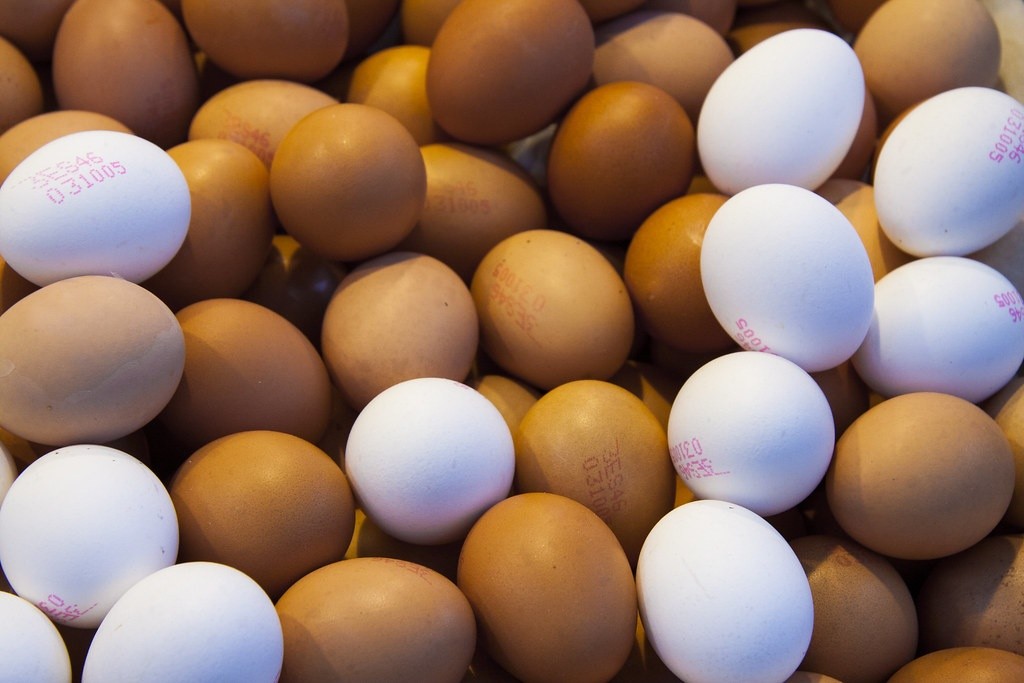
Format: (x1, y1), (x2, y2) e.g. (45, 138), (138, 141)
(0, 0), (1024, 683)
